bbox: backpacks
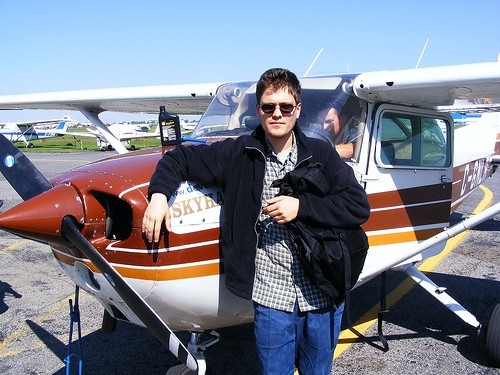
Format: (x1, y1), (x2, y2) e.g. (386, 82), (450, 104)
(270, 162), (369, 299)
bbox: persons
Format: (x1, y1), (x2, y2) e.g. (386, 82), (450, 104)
(318, 101), (359, 158)
(141, 68), (370, 375)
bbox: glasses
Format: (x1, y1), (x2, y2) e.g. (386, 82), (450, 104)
(259, 104), (296, 113)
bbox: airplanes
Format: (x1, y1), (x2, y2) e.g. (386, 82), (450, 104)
(0, 61), (500, 375)
(57, 124), (162, 153)
(0, 118), (78, 148)
